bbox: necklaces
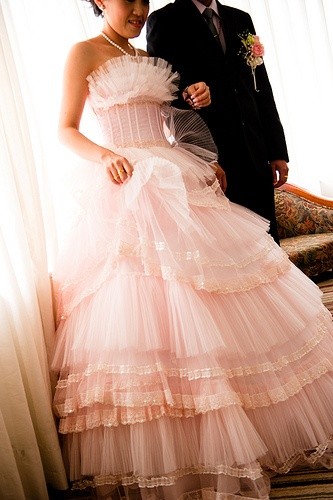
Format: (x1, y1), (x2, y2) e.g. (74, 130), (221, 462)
(99, 30), (139, 61)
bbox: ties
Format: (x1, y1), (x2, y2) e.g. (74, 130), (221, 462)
(203, 8), (224, 54)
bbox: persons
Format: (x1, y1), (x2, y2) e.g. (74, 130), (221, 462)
(146, 0), (289, 247)
(46, 0), (332, 500)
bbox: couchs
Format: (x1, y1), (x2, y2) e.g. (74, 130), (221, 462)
(275, 182), (333, 278)
